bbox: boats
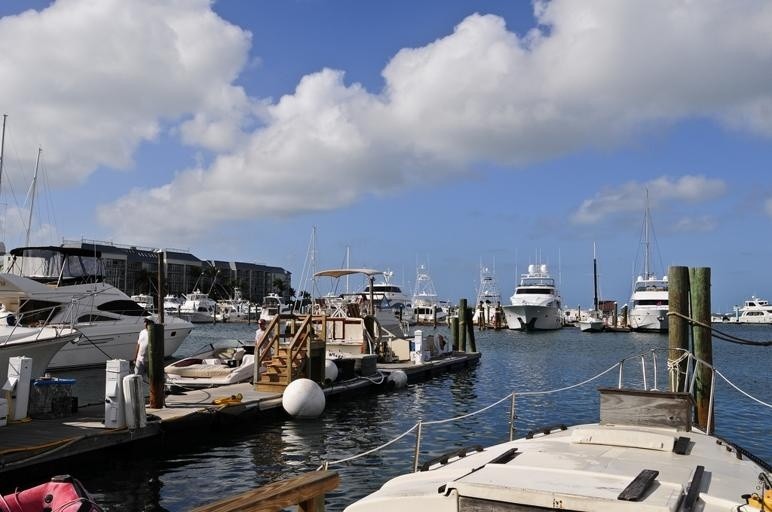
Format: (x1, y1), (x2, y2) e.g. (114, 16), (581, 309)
(0, 474), (100, 512)
(499, 247), (567, 332)
(333, 345), (772, 511)
(712, 295), (771, 326)
(162, 336), (272, 390)
(132, 224), (505, 335)
(0, 276), (89, 400)
(578, 315), (604, 333)
(0, 268), (195, 370)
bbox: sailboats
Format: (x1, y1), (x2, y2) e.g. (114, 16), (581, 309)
(1, 114), (103, 290)
(624, 186), (672, 333)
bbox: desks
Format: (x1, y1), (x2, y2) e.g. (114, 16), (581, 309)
(31, 377), (77, 419)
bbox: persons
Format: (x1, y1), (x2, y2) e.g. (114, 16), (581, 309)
(6, 314), (22, 327)
(254, 319), (276, 369)
(132, 318), (154, 382)
